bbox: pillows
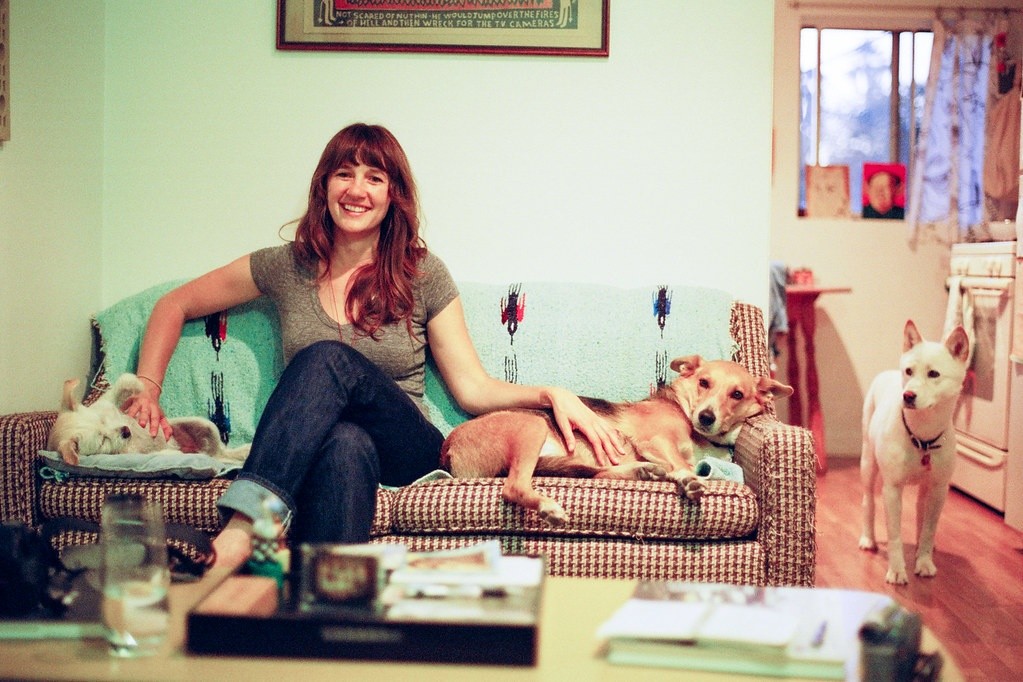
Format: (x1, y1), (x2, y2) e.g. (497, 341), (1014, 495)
(40, 449), (232, 477)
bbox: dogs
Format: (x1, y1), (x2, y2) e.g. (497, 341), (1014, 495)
(44, 373), (253, 467)
(440, 354), (794, 526)
(858, 320), (969, 586)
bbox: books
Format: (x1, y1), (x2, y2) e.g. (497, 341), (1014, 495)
(611, 579), (899, 679)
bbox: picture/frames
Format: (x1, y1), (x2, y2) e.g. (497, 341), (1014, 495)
(275, 0), (611, 58)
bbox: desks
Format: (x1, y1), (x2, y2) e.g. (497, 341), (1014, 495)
(780, 286), (853, 476)
(0, 585), (959, 682)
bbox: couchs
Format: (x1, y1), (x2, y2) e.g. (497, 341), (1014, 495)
(0, 278), (818, 585)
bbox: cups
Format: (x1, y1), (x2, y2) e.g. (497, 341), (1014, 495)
(100, 498), (173, 660)
(969, 220), (1016, 242)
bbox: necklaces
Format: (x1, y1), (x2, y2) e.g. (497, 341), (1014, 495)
(329, 276), (343, 342)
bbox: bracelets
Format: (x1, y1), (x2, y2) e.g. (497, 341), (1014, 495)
(137, 374), (163, 392)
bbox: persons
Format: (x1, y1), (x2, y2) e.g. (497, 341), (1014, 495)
(864, 170), (904, 218)
(118, 120), (626, 570)
